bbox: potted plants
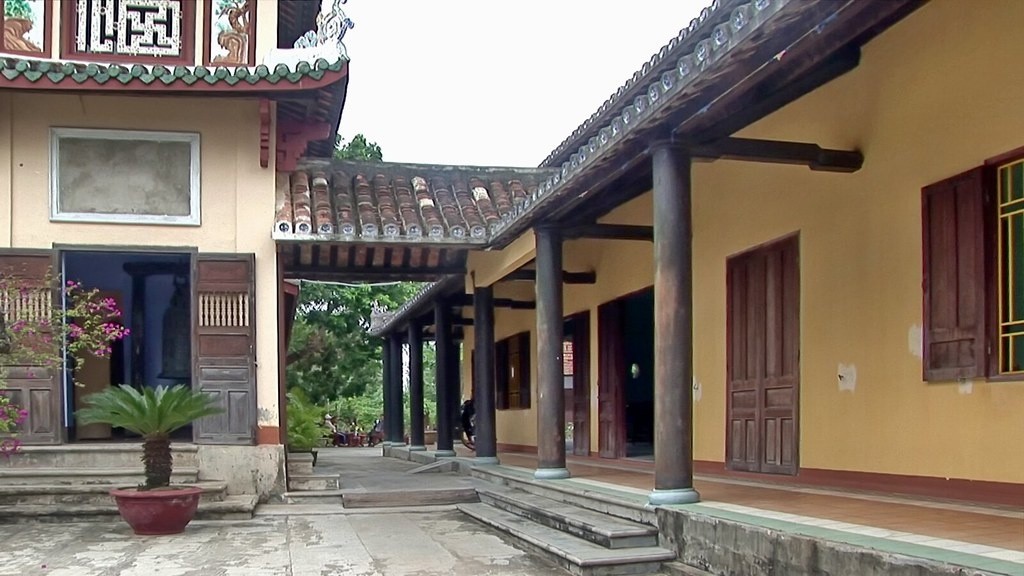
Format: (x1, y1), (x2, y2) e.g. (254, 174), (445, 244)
(284, 387), (324, 467)
(424, 398), (437, 444)
(76, 383), (227, 535)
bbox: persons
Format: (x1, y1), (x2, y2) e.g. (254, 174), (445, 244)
(350, 418), (365, 447)
(325, 414), (348, 446)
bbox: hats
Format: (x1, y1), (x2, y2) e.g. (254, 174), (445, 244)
(325, 414), (332, 418)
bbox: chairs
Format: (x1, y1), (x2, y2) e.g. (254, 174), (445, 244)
(324, 433), (384, 448)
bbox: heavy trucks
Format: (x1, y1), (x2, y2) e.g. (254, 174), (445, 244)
(458, 397), (476, 449)
